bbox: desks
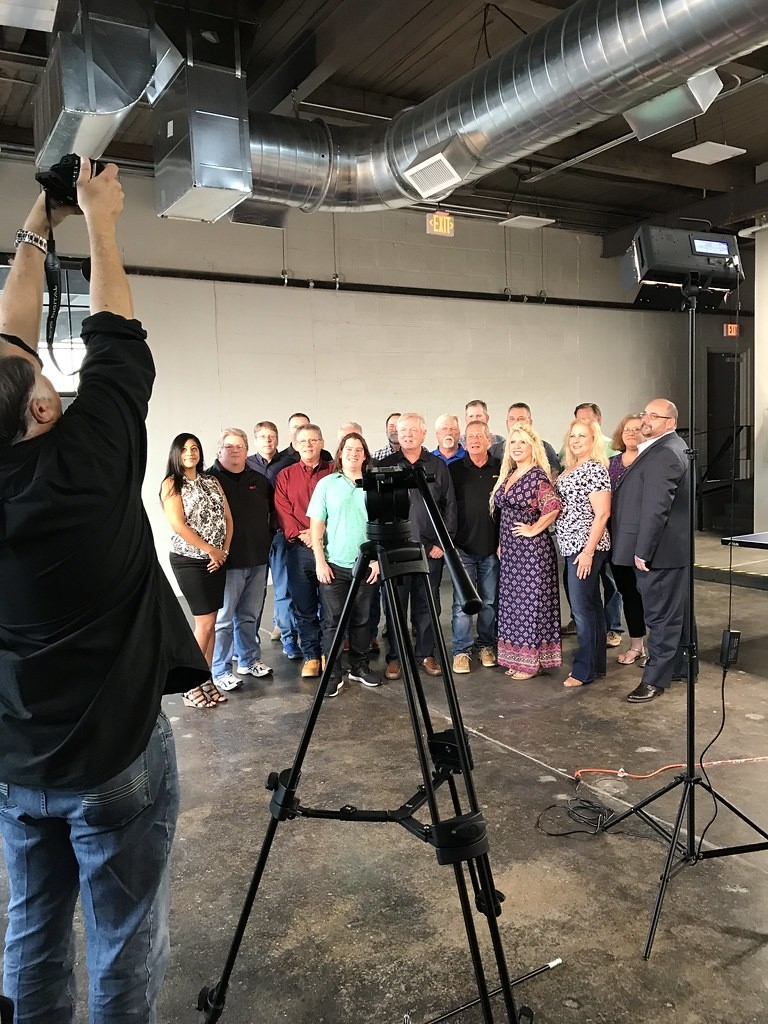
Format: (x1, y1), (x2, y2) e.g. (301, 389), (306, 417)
(721, 532), (768, 550)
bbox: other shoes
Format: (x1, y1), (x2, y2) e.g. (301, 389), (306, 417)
(504, 668), (514, 676)
(510, 667), (543, 679)
(270, 628), (282, 641)
(564, 677), (583, 687)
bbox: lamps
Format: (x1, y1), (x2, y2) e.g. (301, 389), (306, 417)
(673, 102), (747, 166)
(501, 166), (556, 230)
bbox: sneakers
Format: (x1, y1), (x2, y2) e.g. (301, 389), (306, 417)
(301, 659), (320, 677)
(283, 643), (305, 660)
(348, 666), (382, 686)
(237, 661), (273, 677)
(325, 662), (344, 697)
(606, 631), (622, 646)
(561, 620), (577, 634)
(477, 646), (499, 666)
(453, 653), (470, 673)
(215, 674), (244, 691)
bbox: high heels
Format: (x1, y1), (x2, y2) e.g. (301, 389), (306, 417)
(202, 678), (229, 703)
(182, 685), (215, 708)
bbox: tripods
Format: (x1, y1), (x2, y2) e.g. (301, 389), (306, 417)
(196, 456), (533, 1024)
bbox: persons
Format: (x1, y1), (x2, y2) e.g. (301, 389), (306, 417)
(1, 155), (212, 1024)
(158, 398), (699, 709)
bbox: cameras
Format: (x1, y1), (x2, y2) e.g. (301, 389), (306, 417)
(35, 153), (106, 207)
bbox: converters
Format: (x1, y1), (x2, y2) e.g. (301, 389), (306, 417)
(720, 629), (740, 665)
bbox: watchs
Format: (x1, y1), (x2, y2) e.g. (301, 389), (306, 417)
(13, 229), (47, 254)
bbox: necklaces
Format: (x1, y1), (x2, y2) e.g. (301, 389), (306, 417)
(515, 462), (533, 478)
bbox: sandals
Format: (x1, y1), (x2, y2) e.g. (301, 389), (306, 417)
(618, 645), (646, 665)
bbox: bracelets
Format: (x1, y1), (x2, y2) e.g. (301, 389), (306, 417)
(582, 549), (594, 557)
(225, 549), (229, 555)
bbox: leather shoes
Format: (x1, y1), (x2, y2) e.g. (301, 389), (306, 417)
(672, 666), (699, 682)
(368, 636), (381, 652)
(342, 637), (349, 651)
(626, 681), (665, 703)
(422, 656), (442, 675)
(385, 660), (401, 679)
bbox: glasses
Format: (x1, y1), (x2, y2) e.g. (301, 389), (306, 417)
(295, 438), (322, 446)
(623, 426), (641, 433)
(640, 412), (673, 419)
